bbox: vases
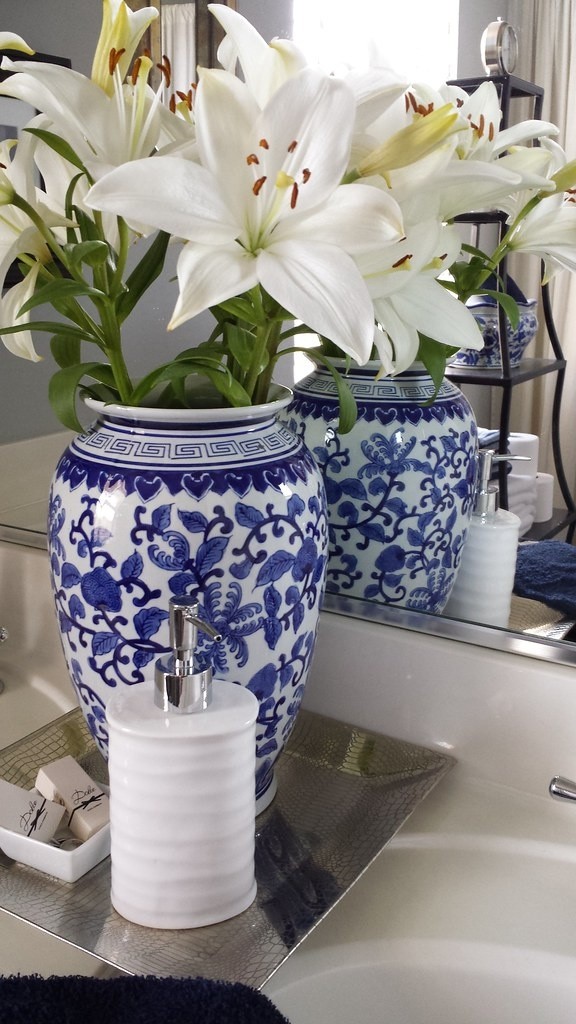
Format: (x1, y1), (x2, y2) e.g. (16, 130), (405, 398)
(442, 221), (538, 369)
(47, 376), (327, 816)
(274, 344), (478, 615)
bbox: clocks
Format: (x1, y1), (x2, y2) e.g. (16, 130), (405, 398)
(480, 17), (518, 77)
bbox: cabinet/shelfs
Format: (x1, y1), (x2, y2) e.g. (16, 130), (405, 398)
(437, 76), (575, 545)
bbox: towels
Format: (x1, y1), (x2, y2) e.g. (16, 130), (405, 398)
(0, 976), (291, 1023)
(514, 541), (575, 618)
(478, 427), (512, 481)
(493, 473), (538, 540)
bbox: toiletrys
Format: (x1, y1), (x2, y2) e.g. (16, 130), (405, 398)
(106, 597), (257, 930)
(445, 451), (532, 628)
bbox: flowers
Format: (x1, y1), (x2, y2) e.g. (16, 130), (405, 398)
(277, 78), (574, 406)
(0, 0), (406, 433)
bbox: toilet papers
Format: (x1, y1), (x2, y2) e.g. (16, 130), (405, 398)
(508, 431), (553, 522)
